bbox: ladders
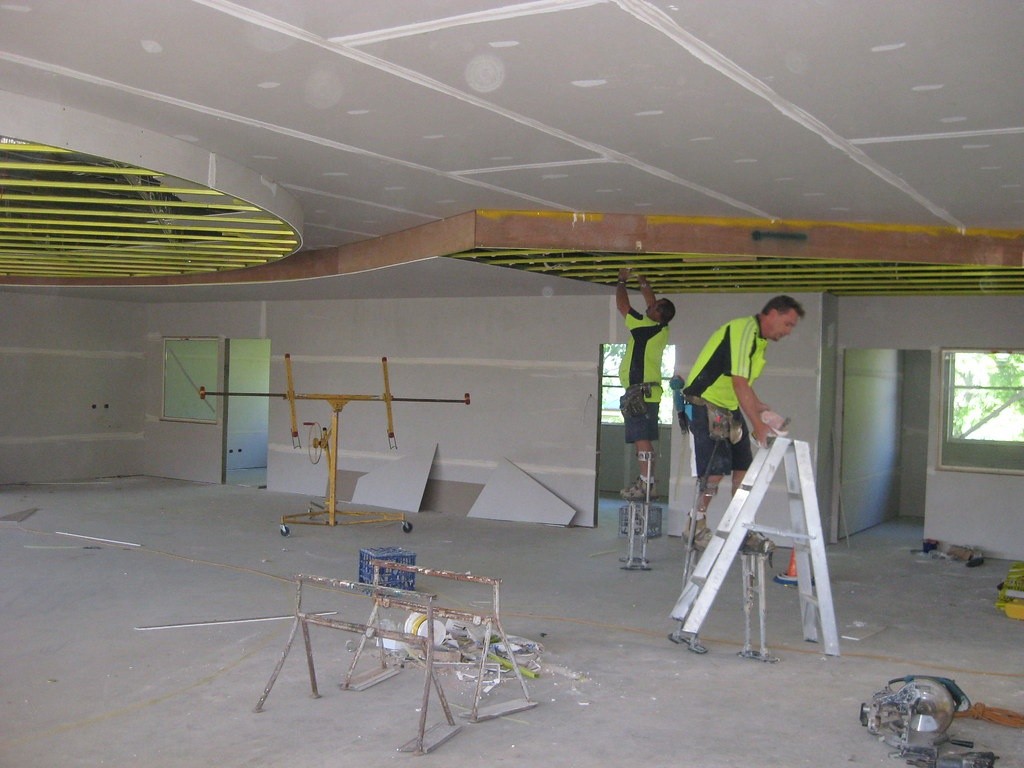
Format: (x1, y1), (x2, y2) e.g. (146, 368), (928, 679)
(670, 435), (842, 658)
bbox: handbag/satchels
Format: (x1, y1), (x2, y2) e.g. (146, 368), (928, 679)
(620, 385), (647, 417)
(684, 396), (742, 445)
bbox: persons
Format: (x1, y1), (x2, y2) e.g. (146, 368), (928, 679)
(617, 268), (675, 502)
(684, 296), (804, 555)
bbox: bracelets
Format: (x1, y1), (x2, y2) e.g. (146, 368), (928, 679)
(641, 283), (647, 288)
(618, 280), (625, 284)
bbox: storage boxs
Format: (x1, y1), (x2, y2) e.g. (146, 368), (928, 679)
(619, 504), (662, 539)
(360, 546), (418, 597)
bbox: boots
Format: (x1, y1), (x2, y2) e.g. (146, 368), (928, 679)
(620, 474), (657, 499)
(682, 512), (714, 552)
(740, 529), (774, 554)
